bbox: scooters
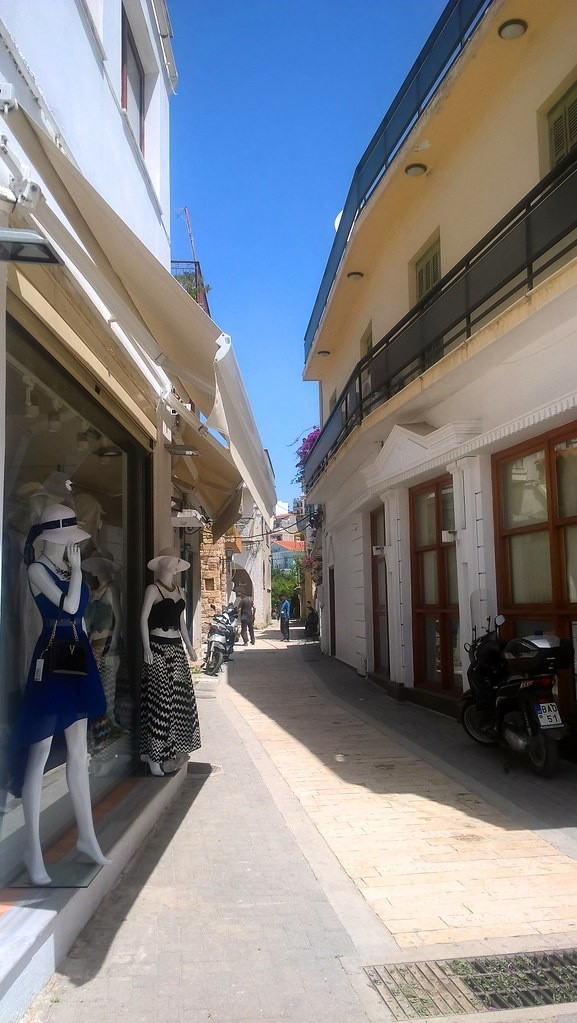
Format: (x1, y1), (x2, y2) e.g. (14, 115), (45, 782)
(299, 600), (319, 639)
(201, 602), (239, 676)
(455, 587), (573, 779)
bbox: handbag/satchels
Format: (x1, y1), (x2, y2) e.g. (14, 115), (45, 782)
(37, 591), (88, 676)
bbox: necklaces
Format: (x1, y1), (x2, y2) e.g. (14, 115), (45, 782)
(157, 579), (175, 592)
(41, 551), (71, 580)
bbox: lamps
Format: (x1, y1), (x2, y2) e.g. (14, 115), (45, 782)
(498, 18), (528, 41)
(347, 271), (364, 280)
(22, 393), (40, 419)
(405, 163), (427, 177)
(318, 351), (330, 357)
(164, 443), (202, 457)
(442, 530), (464, 543)
(76, 432), (89, 452)
(372, 546), (391, 555)
(0, 227), (66, 266)
(48, 410), (62, 432)
(171, 496), (184, 513)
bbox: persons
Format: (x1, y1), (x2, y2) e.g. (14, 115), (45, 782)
(20, 505), (113, 883)
(277, 594), (290, 641)
(81, 550), (122, 748)
(237, 591), (256, 646)
(139, 547), (202, 775)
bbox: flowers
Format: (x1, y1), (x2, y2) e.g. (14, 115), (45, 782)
(285, 425), (321, 484)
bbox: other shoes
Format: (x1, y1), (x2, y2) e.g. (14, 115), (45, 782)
(250, 641), (255, 645)
(281, 638), (289, 642)
(243, 643), (248, 646)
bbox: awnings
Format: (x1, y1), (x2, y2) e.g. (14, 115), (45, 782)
(171, 412), (245, 545)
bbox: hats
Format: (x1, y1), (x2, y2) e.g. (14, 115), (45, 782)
(81, 549), (124, 578)
(6, 483), (63, 523)
(148, 548), (190, 574)
(23, 504), (91, 569)
